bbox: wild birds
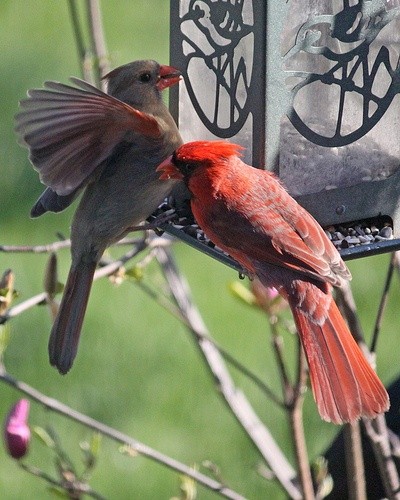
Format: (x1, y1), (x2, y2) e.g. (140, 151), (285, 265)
(15, 60), (185, 376)
(156, 139), (391, 425)
